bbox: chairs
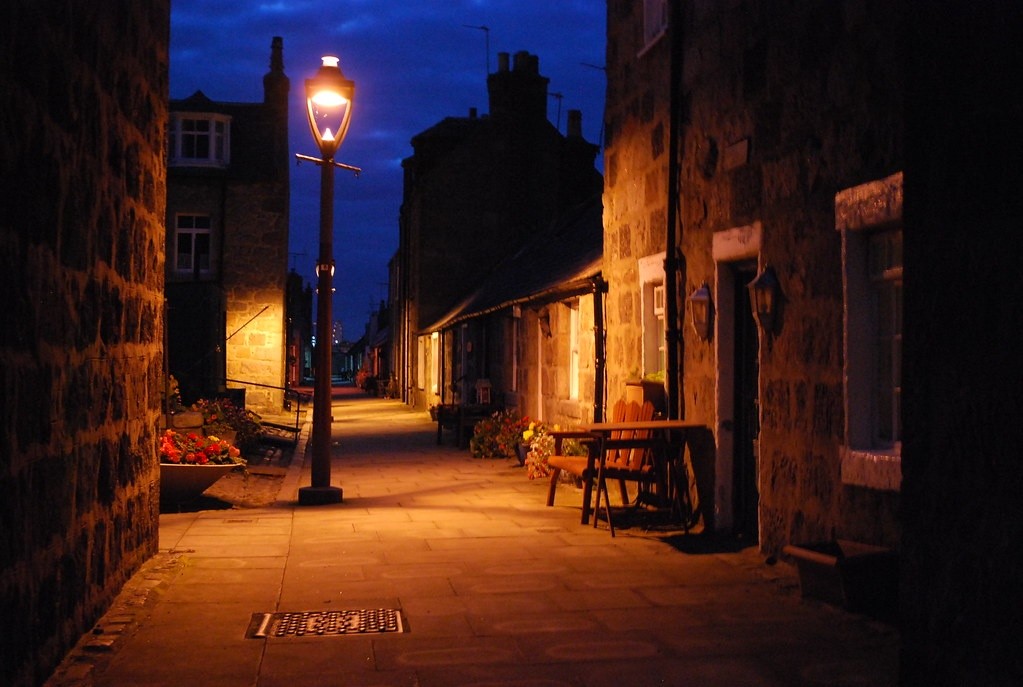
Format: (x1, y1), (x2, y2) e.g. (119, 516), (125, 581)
(546, 398), (663, 527)
(437, 394), (506, 450)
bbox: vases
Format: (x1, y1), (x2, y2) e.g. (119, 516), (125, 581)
(513, 443), (531, 467)
(206, 431), (238, 444)
(159, 463), (241, 506)
(780, 537), (895, 615)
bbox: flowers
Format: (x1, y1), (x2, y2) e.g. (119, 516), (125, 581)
(168, 374), (267, 448)
(468, 409), (589, 484)
(158, 430), (248, 465)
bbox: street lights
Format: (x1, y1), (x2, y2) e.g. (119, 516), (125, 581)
(290, 54), (363, 506)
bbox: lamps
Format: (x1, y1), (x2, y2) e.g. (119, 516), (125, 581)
(745, 262), (789, 352)
(687, 280), (711, 345)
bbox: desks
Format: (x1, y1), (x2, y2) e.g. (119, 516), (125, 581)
(576, 423), (707, 539)
